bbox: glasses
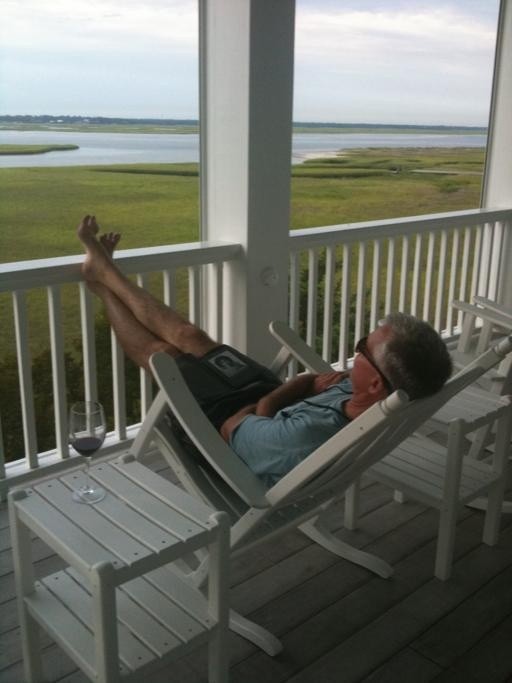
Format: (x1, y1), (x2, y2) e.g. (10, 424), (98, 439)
(355, 336), (392, 394)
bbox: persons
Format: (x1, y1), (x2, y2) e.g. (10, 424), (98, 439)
(78, 215), (453, 493)
(215, 356), (244, 374)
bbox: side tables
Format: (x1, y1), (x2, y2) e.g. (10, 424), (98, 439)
(343, 383), (511, 582)
(5, 449), (235, 681)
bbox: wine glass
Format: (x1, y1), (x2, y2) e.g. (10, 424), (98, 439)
(69, 401), (108, 505)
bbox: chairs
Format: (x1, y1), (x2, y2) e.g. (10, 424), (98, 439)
(131, 295), (512, 657)
